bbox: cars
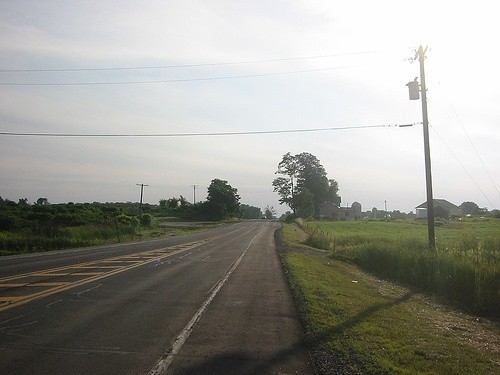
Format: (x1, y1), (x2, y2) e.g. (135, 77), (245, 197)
(262, 216), (266, 219)
